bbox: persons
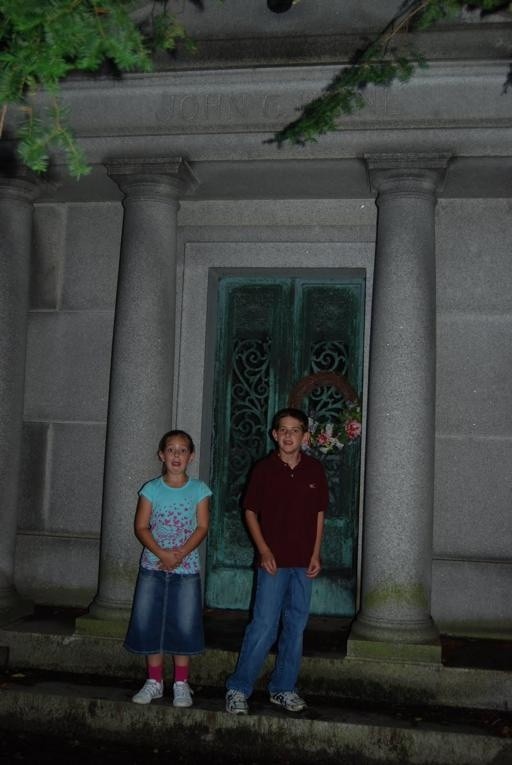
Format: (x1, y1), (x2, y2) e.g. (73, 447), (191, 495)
(222, 407), (331, 716)
(122, 429), (215, 710)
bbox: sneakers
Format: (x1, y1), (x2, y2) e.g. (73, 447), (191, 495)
(132, 679), (164, 704)
(225, 689), (249, 715)
(173, 680), (193, 707)
(270, 691), (307, 711)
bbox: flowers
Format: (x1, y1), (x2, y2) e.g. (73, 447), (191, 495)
(303, 404), (364, 456)
